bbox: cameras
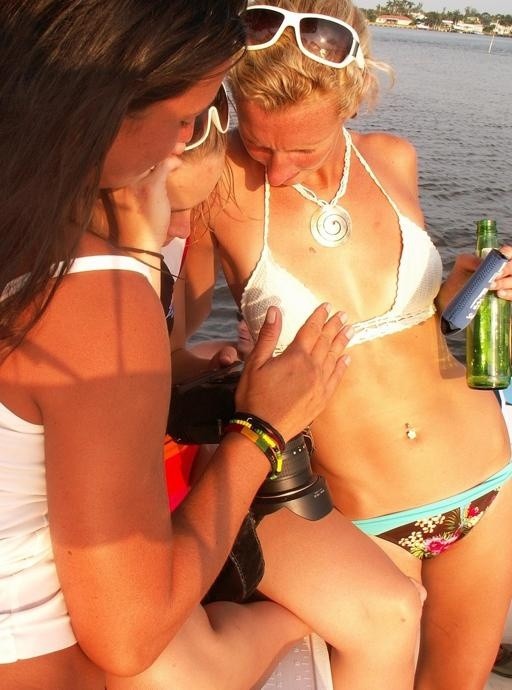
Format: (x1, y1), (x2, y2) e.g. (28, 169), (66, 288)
(165, 355), (333, 522)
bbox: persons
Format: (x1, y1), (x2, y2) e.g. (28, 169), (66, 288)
(0, 0), (352, 690)
(170, 1), (512, 690)
(187, 311), (255, 362)
(105, 80), (427, 690)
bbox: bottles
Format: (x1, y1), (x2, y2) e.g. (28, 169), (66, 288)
(466, 219), (511, 392)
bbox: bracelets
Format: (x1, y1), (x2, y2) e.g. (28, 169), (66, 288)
(224, 413), (286, 480)
(116, 247), (165, 260)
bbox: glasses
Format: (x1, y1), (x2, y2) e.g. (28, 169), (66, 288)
(232, 3), (367, 70)
(182, 80), (232, 151)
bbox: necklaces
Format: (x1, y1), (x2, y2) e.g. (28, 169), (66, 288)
(292, 127), (351, 247)
